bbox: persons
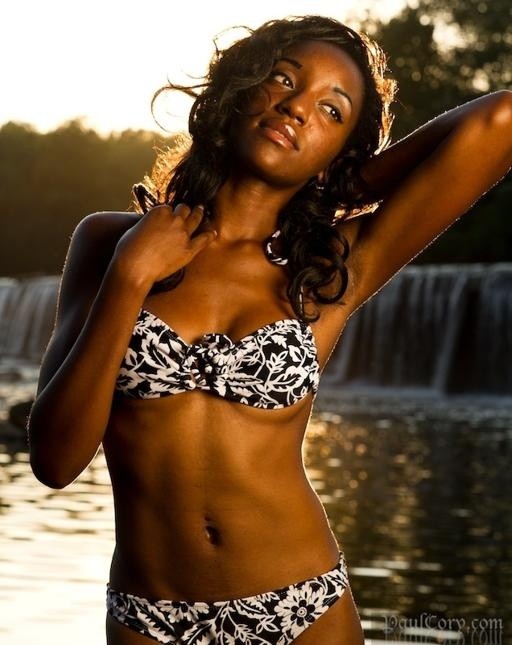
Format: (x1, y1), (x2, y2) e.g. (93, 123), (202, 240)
(28, 14), (511, 644)
